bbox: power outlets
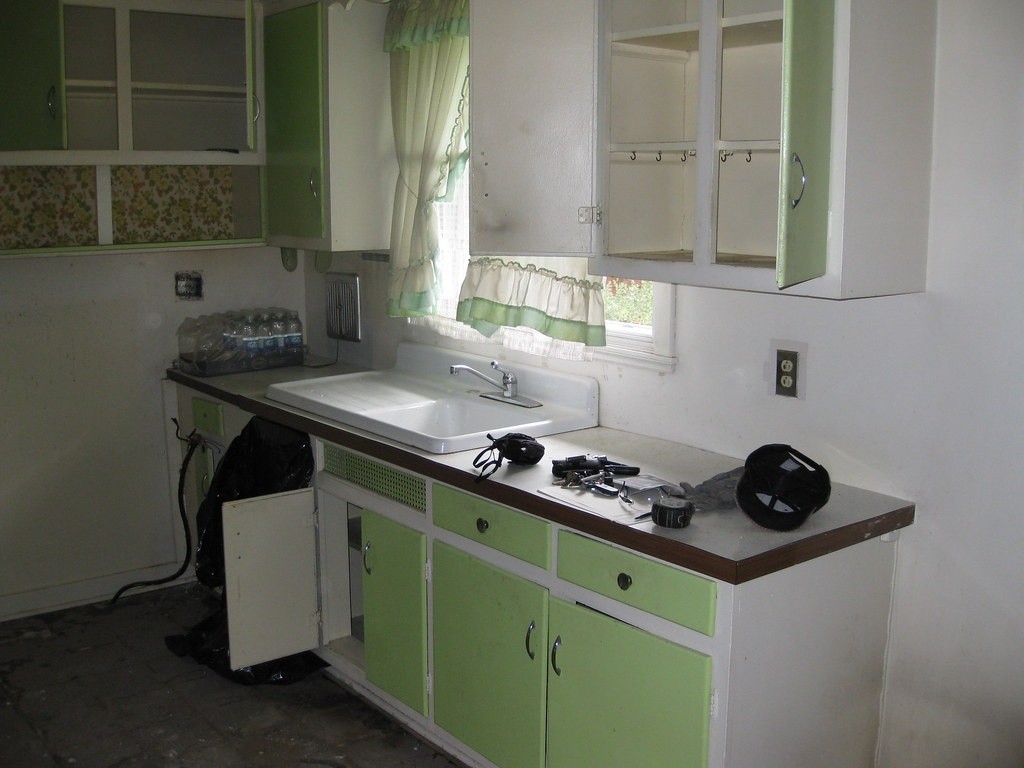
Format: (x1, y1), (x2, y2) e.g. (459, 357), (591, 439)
(763, 336), (809, 402)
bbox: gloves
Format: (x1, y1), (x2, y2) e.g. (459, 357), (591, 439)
(681, 464), (745, 510)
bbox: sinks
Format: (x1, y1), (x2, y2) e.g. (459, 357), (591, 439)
(358, 393), (600, 456)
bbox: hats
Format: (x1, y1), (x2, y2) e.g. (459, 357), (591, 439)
(735, 443), (832, 532)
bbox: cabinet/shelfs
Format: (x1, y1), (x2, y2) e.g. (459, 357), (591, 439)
(0, 0), (269, 259)
(222, 434), (427, 717)
(467, 0), (938, 301)
(173, 384), (256, 594)
(429, 475), (898, 768)
(264, 0), (399, 252)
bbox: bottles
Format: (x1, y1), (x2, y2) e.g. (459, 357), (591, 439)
(222, 307), (304, 370)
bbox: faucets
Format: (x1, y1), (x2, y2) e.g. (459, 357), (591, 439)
(449, 358), (524, 399)
